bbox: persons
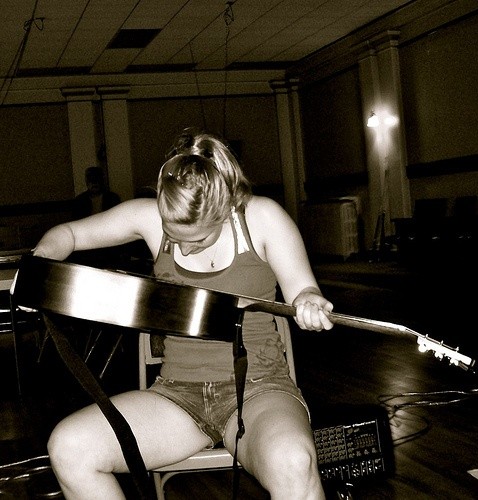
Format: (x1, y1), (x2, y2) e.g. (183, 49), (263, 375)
(72, 166), (122, 222)
(10, 127), (334, 500)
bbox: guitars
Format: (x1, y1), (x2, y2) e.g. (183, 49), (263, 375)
(12, 254), (476, 374)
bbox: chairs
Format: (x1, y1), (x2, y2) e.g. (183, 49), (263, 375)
(138, 315), (298, 500)
(0, 267), (44, 404)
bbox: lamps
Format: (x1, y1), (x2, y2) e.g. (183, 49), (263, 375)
(367, 110), (381, 127)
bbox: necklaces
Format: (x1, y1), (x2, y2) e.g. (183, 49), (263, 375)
(202, 240), (218, 268)
